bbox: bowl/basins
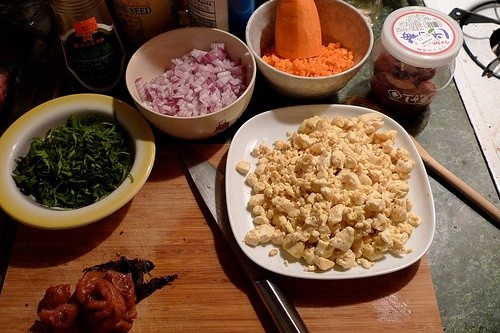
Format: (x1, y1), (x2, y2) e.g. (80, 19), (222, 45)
(125, 26), (256, 141)
(245, 0), (374, 99)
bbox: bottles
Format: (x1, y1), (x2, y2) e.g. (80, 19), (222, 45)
(14, 0), (54, 34)
(175, 0), (218, 27)
(48, 0), (129, 102)
(228, 0), (261, 43)
(113, 0), (176, 50)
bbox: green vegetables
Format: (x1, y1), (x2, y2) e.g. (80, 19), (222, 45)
(12, 116), (135, 206)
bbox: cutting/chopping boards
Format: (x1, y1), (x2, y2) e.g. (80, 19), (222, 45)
(1, 142), (445, 333)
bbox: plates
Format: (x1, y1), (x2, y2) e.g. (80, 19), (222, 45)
(0, 93), (157, 232)
(223, 101), (437, 281)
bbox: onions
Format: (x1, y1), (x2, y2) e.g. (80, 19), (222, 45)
(135, 43), (249, 116)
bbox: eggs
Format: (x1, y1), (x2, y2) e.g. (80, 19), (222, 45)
(236, 111), (421, 273)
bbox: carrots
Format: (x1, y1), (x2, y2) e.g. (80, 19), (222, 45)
(261, 44), (355, 77)
(275, 1), (323, 59)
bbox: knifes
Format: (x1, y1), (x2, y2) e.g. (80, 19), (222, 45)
(173, 140), (308, 332)
